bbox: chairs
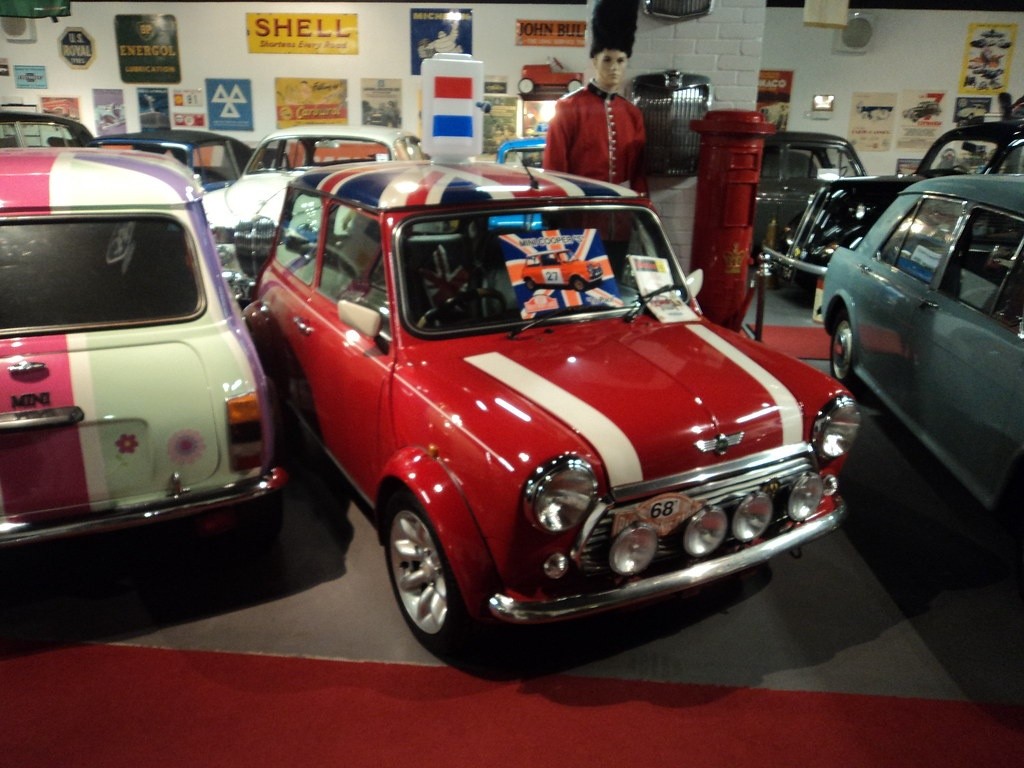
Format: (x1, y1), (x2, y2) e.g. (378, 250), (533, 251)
(406, 232), (473, 315)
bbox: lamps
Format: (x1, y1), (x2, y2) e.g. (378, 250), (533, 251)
(566, 78), (584, 94)
(518, 77), (536, 98)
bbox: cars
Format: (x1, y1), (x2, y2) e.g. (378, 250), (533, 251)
(0, 145), (289, 572)
(0, 103), (434, 312)
(238, 161), (863, 660)
(820, 173), (1023, 572)
(749, 92), (1024, 313)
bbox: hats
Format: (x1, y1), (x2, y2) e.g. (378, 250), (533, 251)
(589, 0), (639, 59)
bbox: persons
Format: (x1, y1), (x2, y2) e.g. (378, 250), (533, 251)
(542, 0), (657, 282)
